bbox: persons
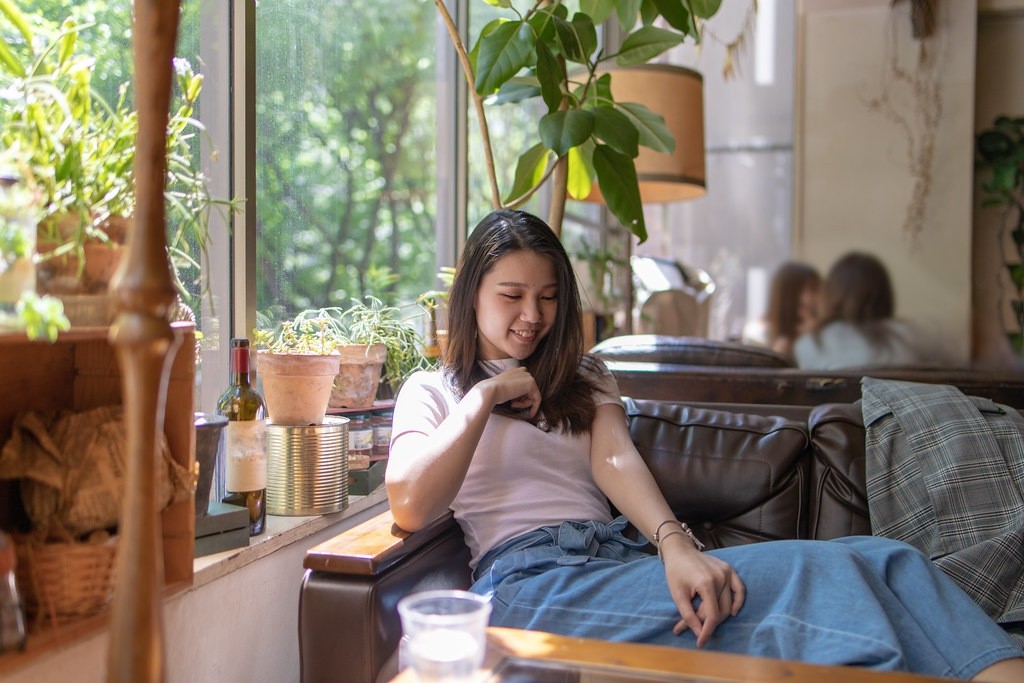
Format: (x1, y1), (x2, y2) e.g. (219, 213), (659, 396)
(792, 253), (948, 371)
(384, 207), (1024, 683)
(763, 260), (836, 355)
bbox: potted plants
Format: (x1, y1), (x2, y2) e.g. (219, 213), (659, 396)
(0, 0), (459, 427)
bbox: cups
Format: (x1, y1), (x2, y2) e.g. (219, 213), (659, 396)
(1, 534), (26, 651)
(397, 588), (492, 683)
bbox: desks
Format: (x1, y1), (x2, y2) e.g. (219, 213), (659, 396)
(386, 625), (981, 683)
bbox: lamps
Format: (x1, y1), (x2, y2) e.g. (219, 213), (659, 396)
(559, 65), (706, 339)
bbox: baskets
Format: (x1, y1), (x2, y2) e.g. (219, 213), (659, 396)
(19, 534), (121, 613)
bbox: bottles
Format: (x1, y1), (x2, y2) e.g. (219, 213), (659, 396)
(214, 338), (269, 536)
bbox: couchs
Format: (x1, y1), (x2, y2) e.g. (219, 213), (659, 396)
(296, 331), (1024, 683)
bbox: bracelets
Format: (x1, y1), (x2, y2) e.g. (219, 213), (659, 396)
(654, 520), (704, 563)
